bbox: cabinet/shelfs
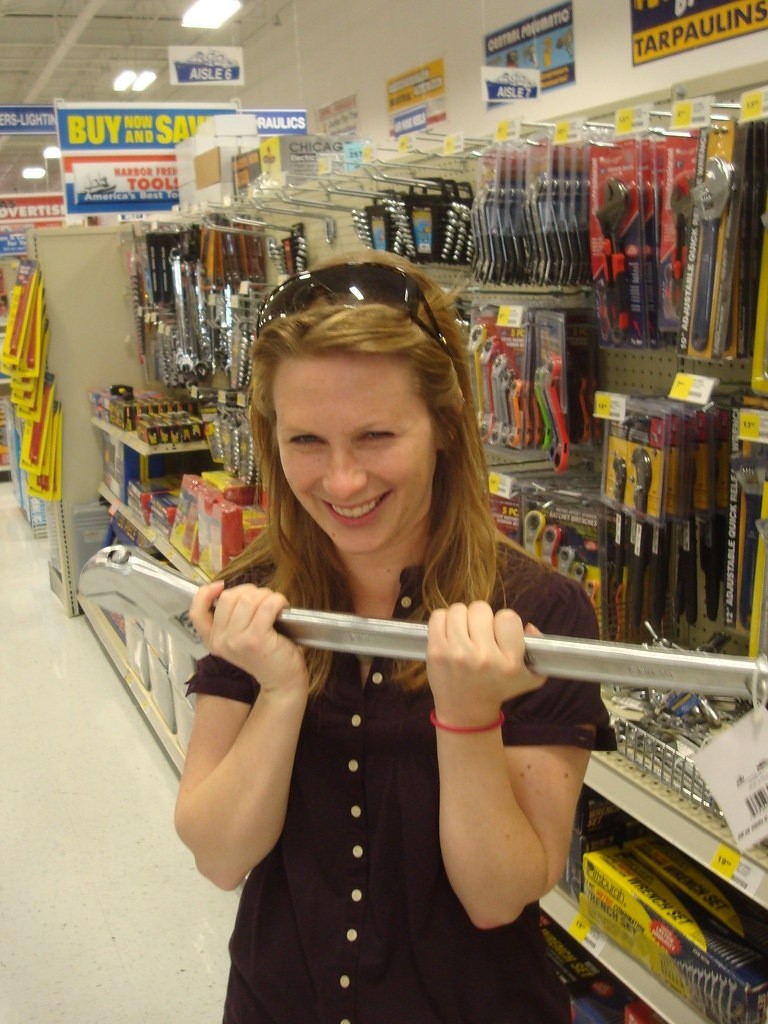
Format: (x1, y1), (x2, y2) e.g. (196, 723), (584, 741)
(0, 252), (28, 473)
(71, 482), (221, 781)
(539, 751), (768, 1024)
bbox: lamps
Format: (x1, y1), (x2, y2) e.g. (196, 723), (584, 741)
(110, 40), (157, 92)
(180, 0), (241, 29)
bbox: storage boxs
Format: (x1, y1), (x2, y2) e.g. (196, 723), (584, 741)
(539, 788), (768, 1024)
(88, 384), (274, 585)
(173, 115), (344, 207)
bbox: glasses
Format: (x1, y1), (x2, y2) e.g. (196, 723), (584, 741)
(256, 262), (455, 360)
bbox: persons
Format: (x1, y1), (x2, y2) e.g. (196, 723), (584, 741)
(173, 248), (615, 1024)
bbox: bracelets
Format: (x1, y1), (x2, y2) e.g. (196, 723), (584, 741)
(430, 709), (504, 733)
